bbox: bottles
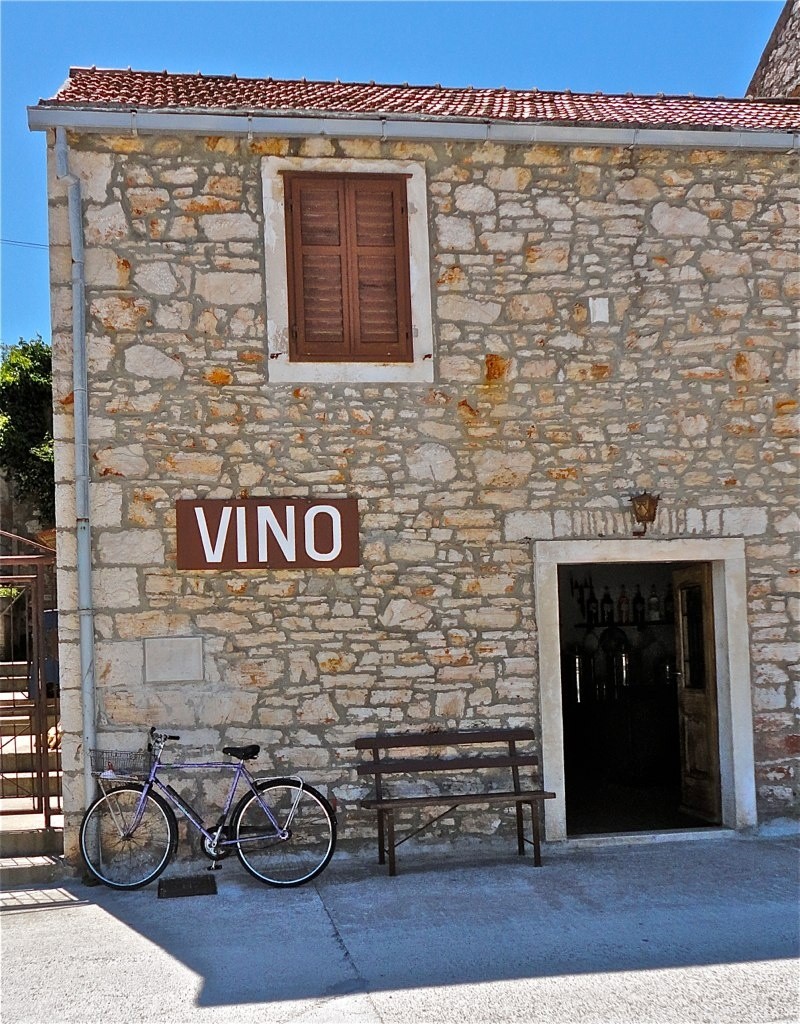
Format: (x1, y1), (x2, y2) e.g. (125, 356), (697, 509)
(587, 586), (599, 624)
(612, 651), (628, 686)
(617, 584), (629, 624)
(574, 652), (595, 704)
(664, 583), (676, 622)
(634, 585), (645, 622)
(601, 586), (614, 624)
(648, 584), (660, 621)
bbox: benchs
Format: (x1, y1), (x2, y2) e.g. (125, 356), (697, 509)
(354, 729), (556, 876)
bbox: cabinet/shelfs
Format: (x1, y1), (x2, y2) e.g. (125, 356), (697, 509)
(577, 621), (680, 798)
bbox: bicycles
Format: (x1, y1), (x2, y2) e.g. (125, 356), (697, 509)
(78, 726), (338, 891)
